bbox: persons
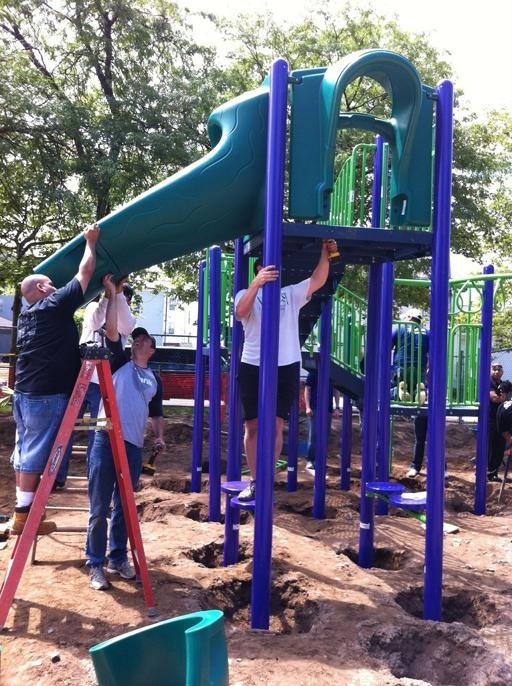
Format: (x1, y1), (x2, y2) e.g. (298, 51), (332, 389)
(9, 221), (106, 538)
(405, 414), (448, 480)
(232, 234), (342, 503)
(86, 271), (167, 590)
(487, 362), (504, 484)
(492, 381), (512, 443)
(303, 369), (341, 469)
(391, 308), (428, 404)
(52, 274), (141, 494)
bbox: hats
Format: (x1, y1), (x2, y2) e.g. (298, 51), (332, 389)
(131, 327), (157, 349)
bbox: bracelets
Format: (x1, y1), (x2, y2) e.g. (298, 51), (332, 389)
(336, 407), (339, 410)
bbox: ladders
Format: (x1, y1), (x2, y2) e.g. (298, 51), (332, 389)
(0, 346), (158, 634)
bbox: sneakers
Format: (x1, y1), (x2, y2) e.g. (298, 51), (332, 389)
(237, 480), (255, 502)
(89, 566), (110, 590)
(399, 381), (410, 401)
(306, 461), (315, 470)
(415, 383), (426, 402)
(489, 476), (502, 483)
(106, 559), (135, 579)
(408, 468), (418, 478)
(9, 511), (58, 535)
